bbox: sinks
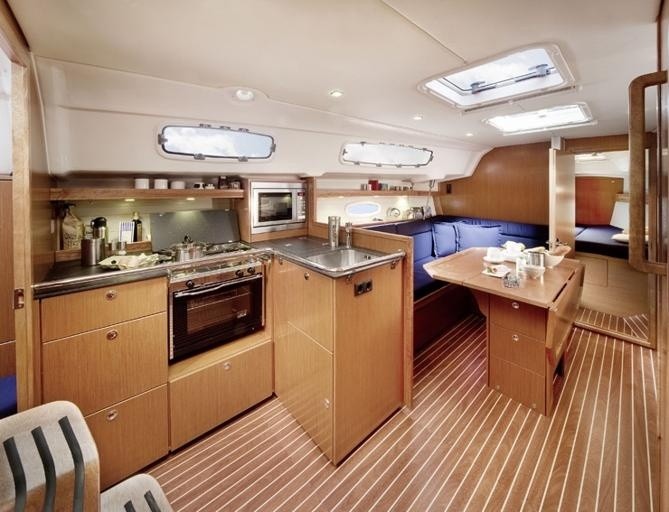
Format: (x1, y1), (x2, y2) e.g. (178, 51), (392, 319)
(307, 250), (381, 270)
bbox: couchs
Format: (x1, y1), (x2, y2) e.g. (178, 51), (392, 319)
(362, 214), (549, 353)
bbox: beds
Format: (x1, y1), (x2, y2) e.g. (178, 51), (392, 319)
(575, 226), (648, 259)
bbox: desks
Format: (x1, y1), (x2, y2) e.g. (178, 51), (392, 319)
(423, 247), (586, 414)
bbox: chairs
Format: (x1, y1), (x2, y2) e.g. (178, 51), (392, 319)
(99, 473), (176, 512)
(0, 401), (101, 512)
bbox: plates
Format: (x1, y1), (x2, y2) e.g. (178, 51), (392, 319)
(483, 256), (506, 263)
(483, 265), (510, 278)
(97, 256), (134, 268)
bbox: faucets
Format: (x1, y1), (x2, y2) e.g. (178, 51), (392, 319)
(343, 222), (354, 248)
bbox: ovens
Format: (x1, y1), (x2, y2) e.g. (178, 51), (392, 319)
(169, 274), (265, 360)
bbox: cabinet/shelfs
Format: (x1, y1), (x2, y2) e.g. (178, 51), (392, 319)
(169, 335), (273, 454)
(272, 234), (406, 466)
(31, 269), (169, 493)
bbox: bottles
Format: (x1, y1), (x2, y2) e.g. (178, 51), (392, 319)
(132, 211), (142, 241)
(218, 176), (228, 189)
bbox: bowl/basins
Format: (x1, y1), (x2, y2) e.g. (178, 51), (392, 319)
(522, 265), (545, 278)
(546, 252), (563, 267)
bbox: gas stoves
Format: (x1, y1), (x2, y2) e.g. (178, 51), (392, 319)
(153, 239), (261, 292)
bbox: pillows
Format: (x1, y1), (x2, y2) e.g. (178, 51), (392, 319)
(432, 220), (468, 256)
(453, 223), (503, 252)
(623, 224), (648, 234)
(612, 234), (648, 244)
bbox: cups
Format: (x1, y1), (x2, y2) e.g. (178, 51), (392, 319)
(488, 248), (505, 259)
